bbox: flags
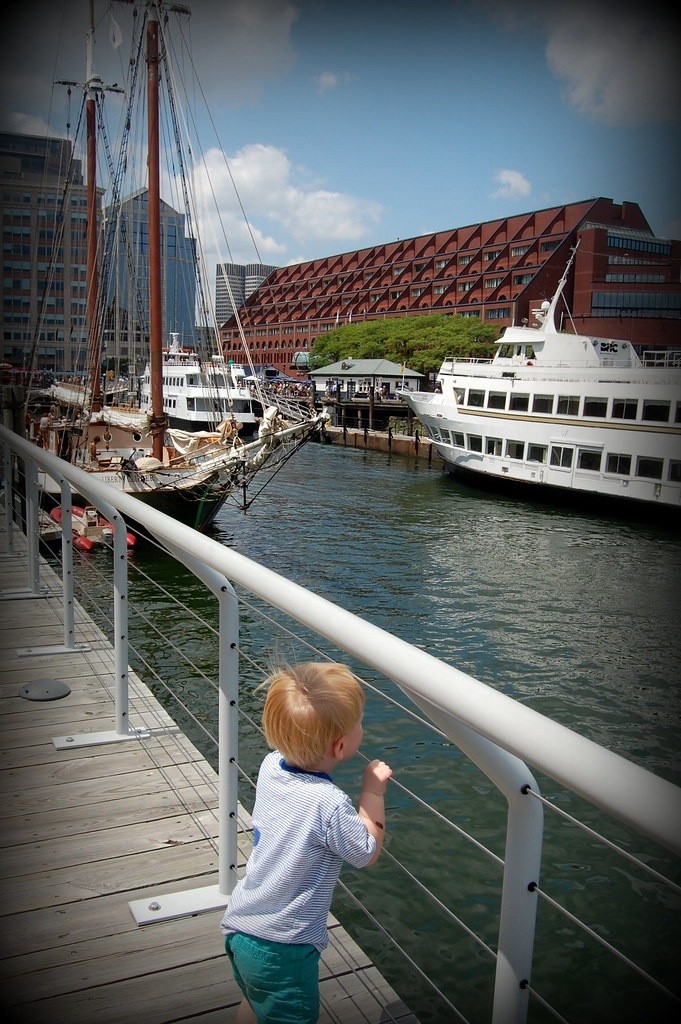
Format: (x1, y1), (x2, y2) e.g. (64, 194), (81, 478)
(400, 365), (406, 374)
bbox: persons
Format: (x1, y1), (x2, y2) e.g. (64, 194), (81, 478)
(237, 378), (315, 398)
(221, 634), (394, 1024)
(14, 371), (88, 387)
(325, 381), (338, 399)
(377, 386), (388, 398)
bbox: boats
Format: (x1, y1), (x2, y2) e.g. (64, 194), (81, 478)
(38, 506), (63, 543)
(139, 333), (257, 436)
(396, 238), (681, 508)
(0, 363), (43, 387)
(45, 372), (130, 407)
(50, 504), (138, 550)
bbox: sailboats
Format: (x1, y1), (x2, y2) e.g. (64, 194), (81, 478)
(15, 0), (333, 535)
